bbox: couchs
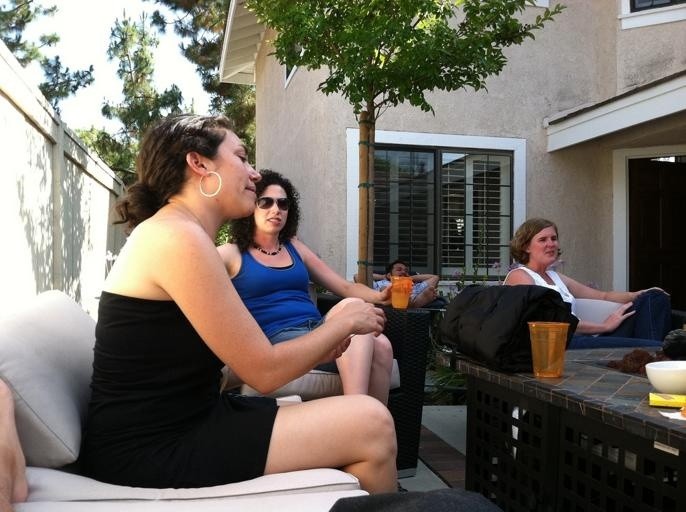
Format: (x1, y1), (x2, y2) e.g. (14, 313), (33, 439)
(0, 283), (432, 512)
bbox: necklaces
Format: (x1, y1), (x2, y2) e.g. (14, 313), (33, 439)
(250, 241), (284, 257)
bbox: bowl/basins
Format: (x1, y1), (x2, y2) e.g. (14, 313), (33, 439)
(644, 359), (686, 396)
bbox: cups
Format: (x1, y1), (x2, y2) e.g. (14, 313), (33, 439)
(526, 320), (570, 379)
(390, 275), (413, 310)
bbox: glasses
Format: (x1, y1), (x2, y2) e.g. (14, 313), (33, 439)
(257, 197), (291, 210)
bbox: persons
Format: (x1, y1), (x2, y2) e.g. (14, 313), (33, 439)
(501, 218), (674, 350)
(354, 261), (449, 313)
(80, 116), (399, 497)
(213, 171), (414, 408)
